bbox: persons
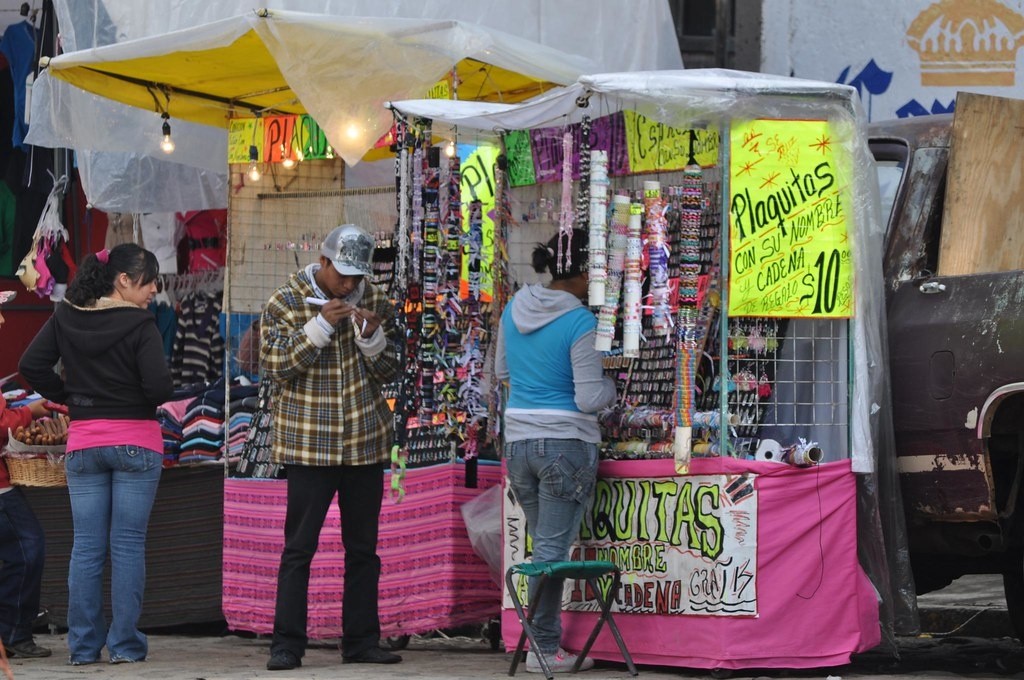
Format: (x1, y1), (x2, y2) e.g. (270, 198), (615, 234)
(0, 291), (52, 658)
(18, 243), (175, 665)
(259, 224), (406, 669)
(496, 228), (617, 672)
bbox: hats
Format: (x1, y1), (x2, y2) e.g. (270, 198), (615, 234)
(0, 290), (17, 304)
(321, 224), (377, 280)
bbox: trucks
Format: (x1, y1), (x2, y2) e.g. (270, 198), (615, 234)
(856, 114), (1023, 638)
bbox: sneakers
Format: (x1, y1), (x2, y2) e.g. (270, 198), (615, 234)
(525, 646), (595, 672)
(267, 655), (302, 671)
(5, 636), (52, 657)
(341, 647), (403, 664)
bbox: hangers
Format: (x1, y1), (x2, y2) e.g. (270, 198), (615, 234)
(154, 266), (225, 306)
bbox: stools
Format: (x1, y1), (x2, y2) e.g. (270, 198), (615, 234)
(506, 560), (637, 680)
